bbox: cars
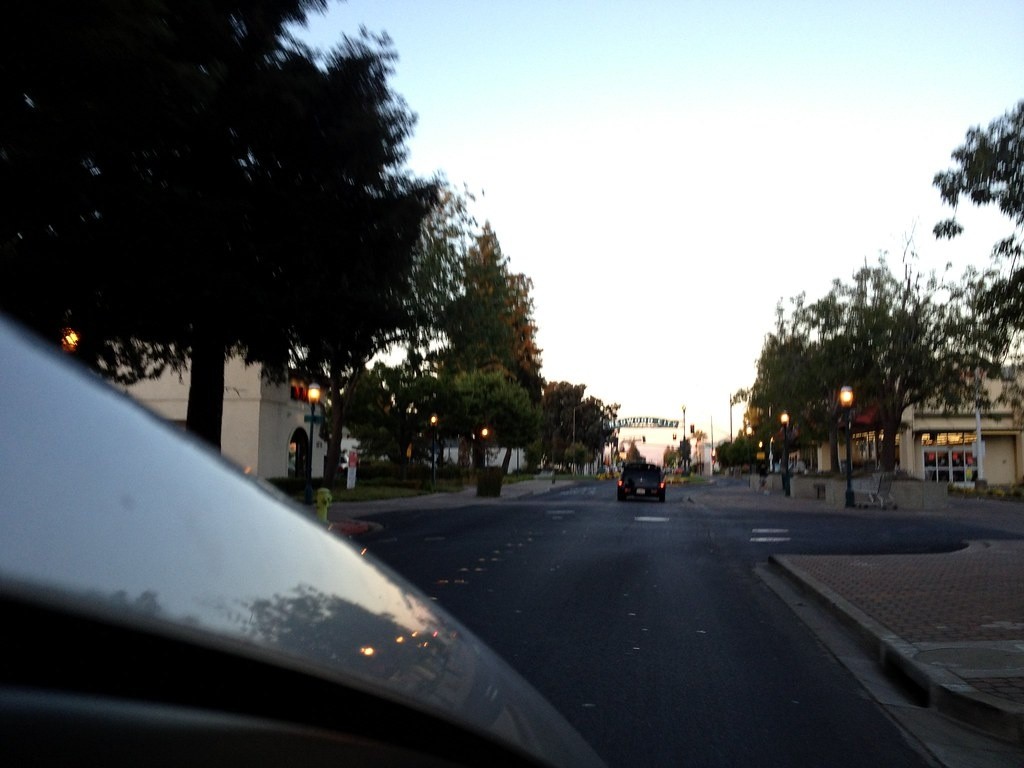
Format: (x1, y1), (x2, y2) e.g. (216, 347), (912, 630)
(618, 462), (669, 503)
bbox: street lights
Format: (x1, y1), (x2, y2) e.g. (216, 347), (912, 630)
(302, 382), (318, 507)
(682, 405), (691, 478)
(781, 412), (792, 499)
(839, 385), (858, 509)
(430, 413), (440, 492)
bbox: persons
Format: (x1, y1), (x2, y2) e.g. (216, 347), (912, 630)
(756, 461), (769, 495)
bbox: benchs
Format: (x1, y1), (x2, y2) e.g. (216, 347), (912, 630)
(812, 481), (854, 501)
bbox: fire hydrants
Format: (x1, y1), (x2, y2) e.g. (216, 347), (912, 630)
(315, 487), (334, 525)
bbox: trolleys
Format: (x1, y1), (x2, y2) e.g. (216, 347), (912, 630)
(859, 471), (898, 511)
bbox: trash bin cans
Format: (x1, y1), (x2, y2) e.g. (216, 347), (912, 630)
(475, 465), (504, 496)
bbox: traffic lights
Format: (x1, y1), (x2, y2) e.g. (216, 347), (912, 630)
(690, 424), (694, 433)
(673, 433), (677, 440)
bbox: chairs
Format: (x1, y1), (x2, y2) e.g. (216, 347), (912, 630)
(852, 472), (899, 510)
